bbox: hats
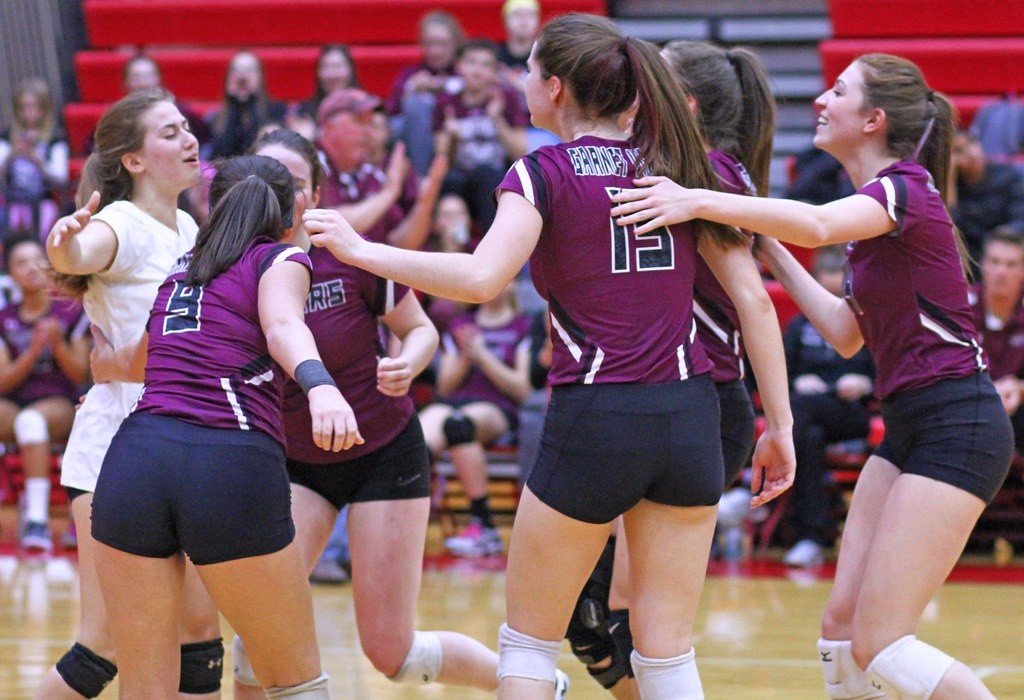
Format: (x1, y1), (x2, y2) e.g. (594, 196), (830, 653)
(316, 88), (382, 122)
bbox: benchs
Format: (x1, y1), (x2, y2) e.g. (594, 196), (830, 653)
(0, 0), (609, 519)
(673, 0), (1024, 499)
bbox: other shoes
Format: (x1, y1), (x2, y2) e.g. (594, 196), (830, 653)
(22, 522), (54, 553)
(444, 518), (504, 557)
(309, 562), (348, 581)
(61, 523), (78, 547)
(718, 488), (772, 525)
(783, 540), (826, 568)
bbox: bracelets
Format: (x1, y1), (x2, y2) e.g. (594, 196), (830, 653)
(294, 359), (338, 396)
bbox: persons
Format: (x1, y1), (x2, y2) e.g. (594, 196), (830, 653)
(0, 0), (1024, 571)
(89, 156), (365, 700)
(36, 91), (223, 699)
(74, 131), (570, 700)
(609, 53), (1015, 700)
(299, 13), (796, 700)
(569, 38), (777, 700)
